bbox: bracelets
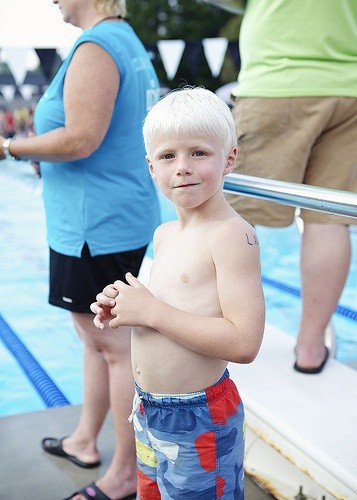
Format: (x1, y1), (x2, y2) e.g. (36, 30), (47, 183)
(3, 137), (16, 161)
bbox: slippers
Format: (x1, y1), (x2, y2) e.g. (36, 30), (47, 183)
(293, 344), (330, 374)
(42, 436), (101, 469)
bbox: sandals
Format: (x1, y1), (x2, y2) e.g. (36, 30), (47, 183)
(64, 481), (137, 500)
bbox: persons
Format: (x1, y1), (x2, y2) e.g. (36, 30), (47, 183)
(90, 85), (266, 500)
(0, 0), (164, 500)
(223, 0), (357, 376)
